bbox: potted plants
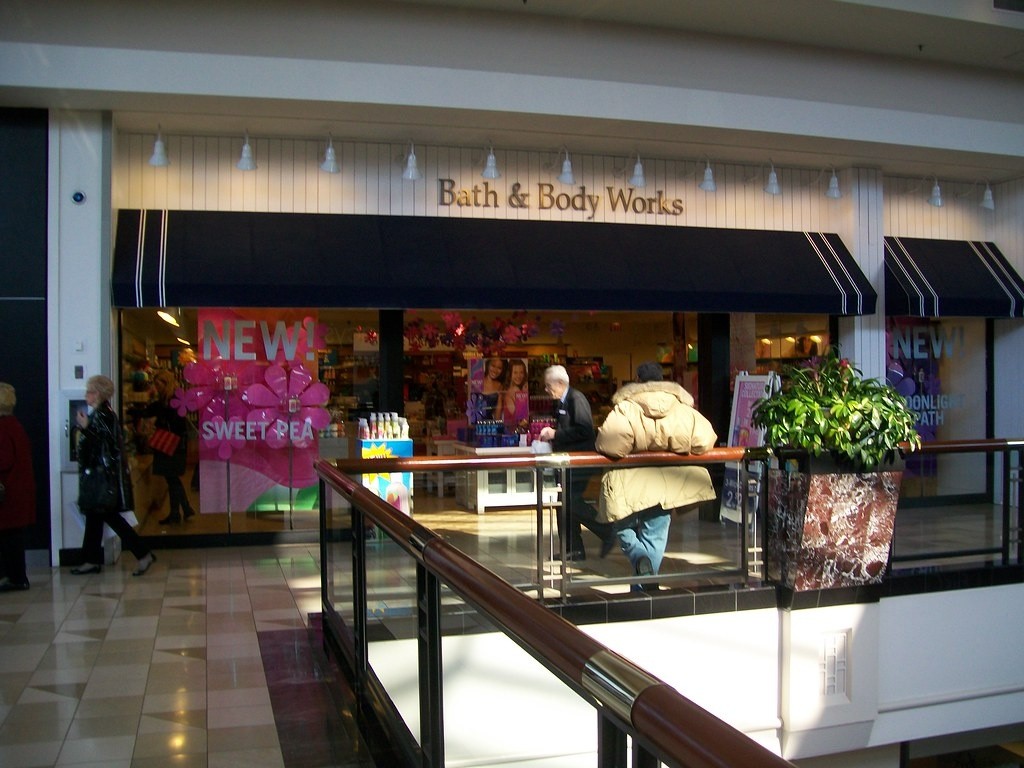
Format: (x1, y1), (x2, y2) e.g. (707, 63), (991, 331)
(753, 355), (925, 592)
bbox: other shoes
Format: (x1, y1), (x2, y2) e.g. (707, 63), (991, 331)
(601, 523), (617, 557)
(184, 504), (195, 519)
(549, 547), (586, 561)
(635, 555), (659, 592)
(159, 512), (181, 525)
(0, 575), (31, 592)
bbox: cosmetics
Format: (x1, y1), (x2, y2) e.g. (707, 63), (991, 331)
(474, 418), (558, 447)
(358, 412), (410, 439)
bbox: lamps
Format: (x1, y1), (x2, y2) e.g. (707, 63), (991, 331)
(157, 307), (180, 327)
(907, 172), (944, 207)
(812, 165), (842, 200)
(321, 131), (341, 174)
(397, 139), (422, 181)
(177, 335), (191, 345)
(474, 141), (500, 179)
(957, 177), (994, 208)
(614, 148), (646, 186)
(686, 154), (717, 190)
(149, 123), (171, 167)
(748, 159), (782, 194)
(548, 144), (577, 185)
(236, 130), (256, 171)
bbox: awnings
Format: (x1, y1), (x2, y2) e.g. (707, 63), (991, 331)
(883, 236), (1024, 318)
(111, 207), (878, 317)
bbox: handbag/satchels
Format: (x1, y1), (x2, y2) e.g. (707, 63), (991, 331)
(147, 410), (182, 457)
(532, 433), (556, 472)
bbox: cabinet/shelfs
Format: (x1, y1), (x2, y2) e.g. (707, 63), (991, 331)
(456, 445), (562, 515)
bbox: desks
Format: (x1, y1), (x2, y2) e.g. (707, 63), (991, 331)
(422, 438), (466, 498)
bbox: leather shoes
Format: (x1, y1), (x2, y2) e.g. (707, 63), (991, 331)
(70, 564), (101, 574)
(133, 554), (156, 575)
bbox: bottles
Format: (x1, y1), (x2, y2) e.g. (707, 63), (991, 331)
(319, 407), (346, 438)
(223, 373), (237, 390)
(289, 394), (300, 413)
(357, 412), (410, 439)
(732, 417), (750, 446)
(527, 419), (557, 446)
(476, 420), (518, 447)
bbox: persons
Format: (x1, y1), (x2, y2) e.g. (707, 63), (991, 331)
(538, 364), (616, 562)
(593, 361), (719, 591)
(69, 375), (158, 577)
(126, 368), (200, 526)
(0, 382), (38, 593)
(479, 358), (528, 426)
